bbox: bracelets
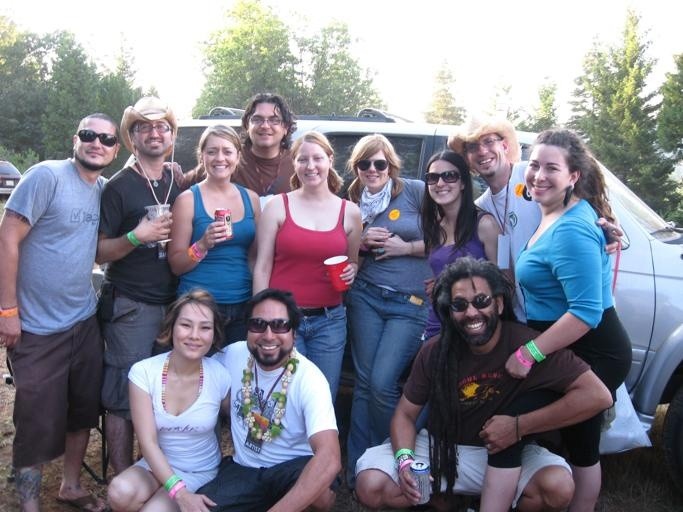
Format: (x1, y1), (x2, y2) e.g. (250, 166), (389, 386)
(407, 240), (414, 255)
(188, 249), (201, 265)
(398, 457), (414, 473)
(0, 305), (19, 318)
(393, 445), (414, 459)
(161, 474), (182, 491)
(515, 346), (535, 368)
(191, 242), (203, 260)
(526, 342), (547, 364)
(127, 230), (146, 248)
(168, 479), (187, 498)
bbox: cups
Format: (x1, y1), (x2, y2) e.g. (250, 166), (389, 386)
(144, 203), (174, 244)
(323, 256), (353, 293)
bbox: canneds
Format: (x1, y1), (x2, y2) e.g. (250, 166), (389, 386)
(214, 208), (233, 240)
(409, 460), (430, 504)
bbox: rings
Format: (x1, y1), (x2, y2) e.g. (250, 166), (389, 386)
(376, 248), (384, 253)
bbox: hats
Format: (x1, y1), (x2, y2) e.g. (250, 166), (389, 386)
(118, 96), (178, 159)
(447, 117), (523, 165)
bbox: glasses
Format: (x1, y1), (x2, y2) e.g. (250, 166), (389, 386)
(464, 138), (503, 153)
(245, 314), (294, 333)
(76, 129), (118, 148)
(424, 170), (465, 185)
(248, 116), (284, 126)
(446, 293), (498, 313)
(355, 159), (390, 172)
(130, 121), (174, 134)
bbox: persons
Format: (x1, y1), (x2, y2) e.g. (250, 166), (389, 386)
(0, 113), (187, 511)
(91, 93), (184, 473)
(249, 129), (364, 407)
(479, 127), (634, 512)
(415, 151), (499, 433)
(445, 114), (625, 326)
(105, 291), (234, 512)
(191, 289), (345, 511)
(180, 90), (314, 206)
(343, 135), (437, 491)
(162, 124), (262, 360)
(346, 255), (613, 511)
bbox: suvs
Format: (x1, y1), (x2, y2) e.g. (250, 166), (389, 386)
(123, 104), (683, 481)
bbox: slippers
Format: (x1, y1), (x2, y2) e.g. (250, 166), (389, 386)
(56, 490), (109, 512)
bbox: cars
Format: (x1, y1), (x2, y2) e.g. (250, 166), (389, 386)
(0, 160), (22, 197)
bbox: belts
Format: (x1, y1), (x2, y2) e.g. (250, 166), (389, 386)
(300, 303), (340, 318)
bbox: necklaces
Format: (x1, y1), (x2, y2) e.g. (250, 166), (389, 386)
(236, 349), (296, 441)
